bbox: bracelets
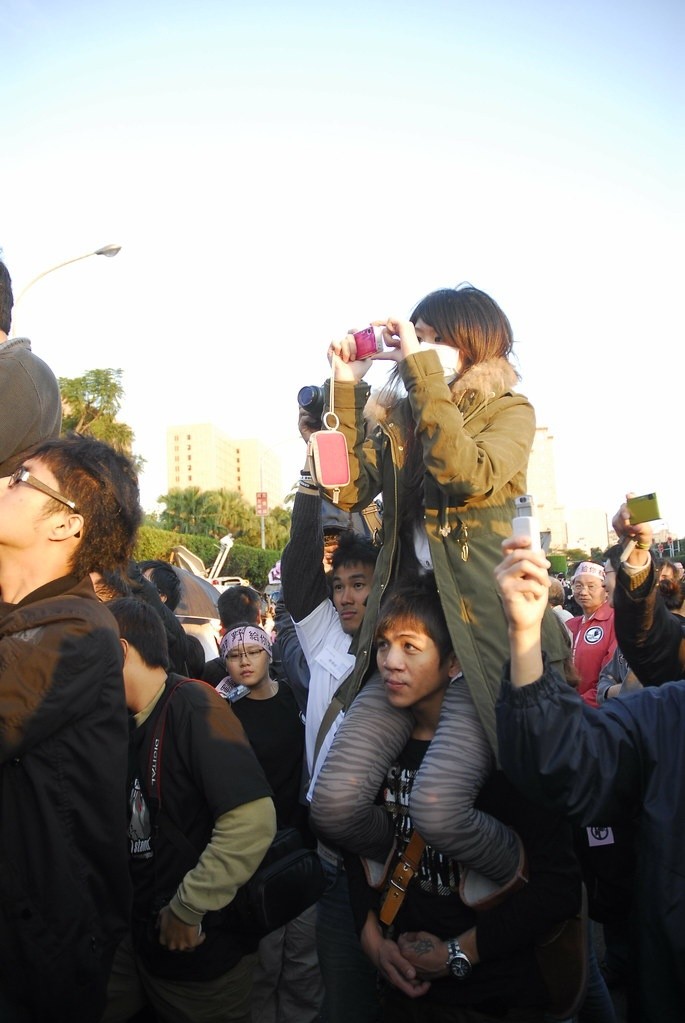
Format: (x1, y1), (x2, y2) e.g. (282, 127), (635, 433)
(299, 470), (310, 476)
(301, 476), (312, 480)
(299, 481), (317, 490)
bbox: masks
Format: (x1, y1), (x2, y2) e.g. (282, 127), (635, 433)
(421, 341), (463, 385)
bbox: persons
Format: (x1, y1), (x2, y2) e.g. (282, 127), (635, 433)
(1, 265), (685, 1022)
(310, 286), (588, 1022)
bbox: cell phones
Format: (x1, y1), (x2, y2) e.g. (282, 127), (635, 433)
(513, 495), (541, 556)
(628, 492), (662, 526)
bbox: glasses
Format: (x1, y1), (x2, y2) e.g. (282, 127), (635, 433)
(8, 467), (81, 537)
(572, 582), (601, 594)
(226, 647), (264, 662)
(602, 570), (615, 580)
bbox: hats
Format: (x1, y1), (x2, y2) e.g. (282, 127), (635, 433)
(0, 263), (14, 333)
(546, 577), (565, 606)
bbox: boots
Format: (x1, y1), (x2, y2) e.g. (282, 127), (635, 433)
(359, 830), (416, 906)
(460, 827), (589, 1020)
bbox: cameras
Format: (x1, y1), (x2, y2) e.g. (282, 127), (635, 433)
(296, 385), (328, 429)
(352, 326), (383, 360)
(222, 685), (251, 707)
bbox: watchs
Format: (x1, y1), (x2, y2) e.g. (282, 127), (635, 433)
(446, 938), (472, 980)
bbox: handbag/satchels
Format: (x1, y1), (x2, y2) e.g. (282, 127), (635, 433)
(306, 350), (351, 504)
(235, 825), (327, 933)
(141, 893), (224, 975)
